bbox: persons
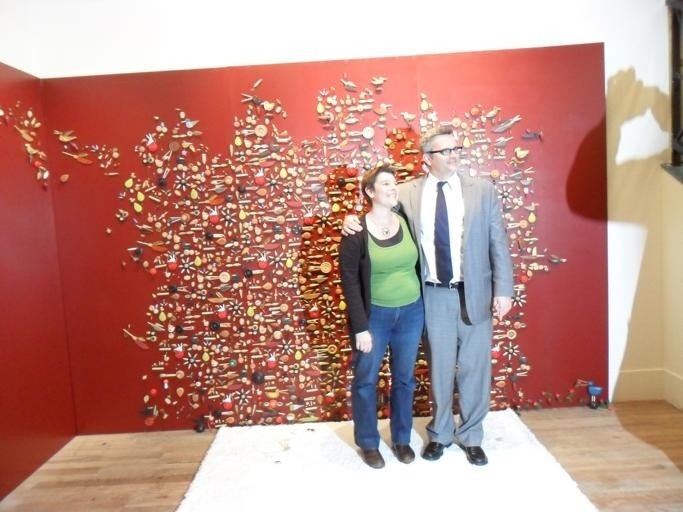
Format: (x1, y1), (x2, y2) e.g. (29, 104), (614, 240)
(337, 163), (427, 469)
(337, 123), (514, 467)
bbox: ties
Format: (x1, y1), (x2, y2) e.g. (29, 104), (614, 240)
(433, 181), (453, 284)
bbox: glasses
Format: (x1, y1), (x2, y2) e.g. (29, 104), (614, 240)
(427, 146), (463, 156)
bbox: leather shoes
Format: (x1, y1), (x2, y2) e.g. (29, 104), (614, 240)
(363, 448), (385, 469)
(422, 441), (452, 461)
(460, 443), (488, 466)
(394, 444), (415, 464)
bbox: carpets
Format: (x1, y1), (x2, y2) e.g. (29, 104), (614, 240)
(173, 407), (601, 512)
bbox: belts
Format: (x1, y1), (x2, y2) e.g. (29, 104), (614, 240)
(425, 281), (464, 289)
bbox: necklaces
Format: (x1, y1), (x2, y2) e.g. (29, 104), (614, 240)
(368, 211), (397, 238)
